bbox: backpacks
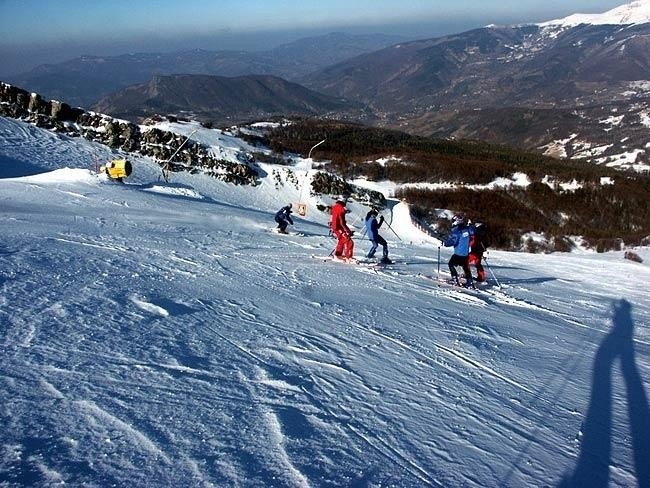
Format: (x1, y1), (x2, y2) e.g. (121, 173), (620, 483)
(474, 222), (491, 250)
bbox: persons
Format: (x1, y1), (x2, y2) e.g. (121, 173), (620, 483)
(463, 217), (489, 282)
(366, 206), (392, 264)
(441, 212), (475, 288)
(275, 202), (293, 233)
(331, 194), (354, 259)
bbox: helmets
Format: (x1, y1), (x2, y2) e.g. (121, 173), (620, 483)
(463, 217), (471, 227)
(451, 215), (462, 225)
(338, 195), (346, 203)
(288, 203), (293, 207)
(372, 207), (379, 213)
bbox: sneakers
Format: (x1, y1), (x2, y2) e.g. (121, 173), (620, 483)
(334, 256), (344, 262)
(281, 231), (288, 234)
(366, 255), (375, 257)
(448, 278), (460, 285)
(383, 257), (395, 264)
(474, 276), (484, 282)
(462, 282), (471, 287)
(346, 257), (357, 263)
